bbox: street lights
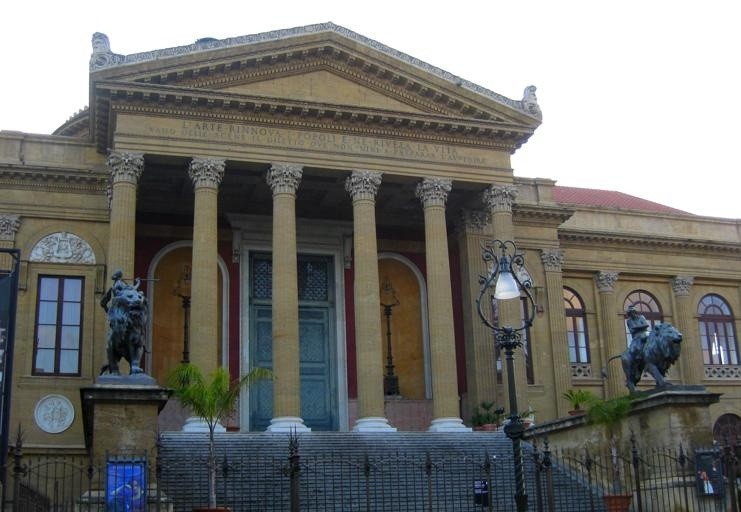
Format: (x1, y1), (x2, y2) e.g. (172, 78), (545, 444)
(381, 279), (401, 396)
(476, 237), (537, 511)
(174, 265), (191, 364)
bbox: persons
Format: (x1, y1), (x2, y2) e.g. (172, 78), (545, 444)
(626, 305), (651, 385)
(114, 277), (152, 354)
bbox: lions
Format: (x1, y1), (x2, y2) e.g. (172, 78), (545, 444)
(100, 285), (150, 377)
(607, 322), (684, 394)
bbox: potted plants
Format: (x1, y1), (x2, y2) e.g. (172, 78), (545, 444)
(469, 399), (500, 432)
(560, 389), (601, 416)
(520, 409), (537, 428)
(165, 360), (280, 511)
(588, 393), (649, 511)
